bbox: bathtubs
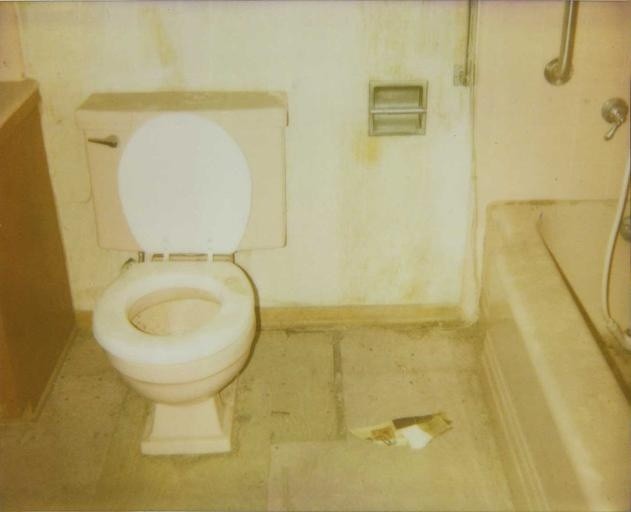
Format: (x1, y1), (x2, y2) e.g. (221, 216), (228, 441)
(481, 200), (631, 512)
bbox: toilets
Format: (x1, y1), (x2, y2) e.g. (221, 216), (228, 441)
(72, 90), (289, 457)
